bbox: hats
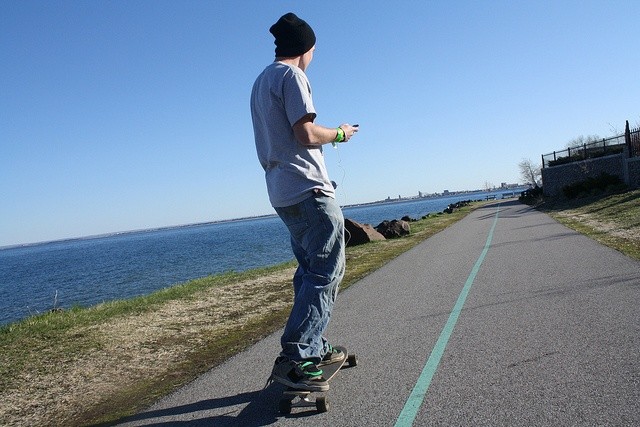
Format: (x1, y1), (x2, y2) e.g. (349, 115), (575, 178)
(270, 13), (316, 57)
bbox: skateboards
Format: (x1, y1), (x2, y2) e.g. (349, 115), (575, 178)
(279, 346), (358, 414)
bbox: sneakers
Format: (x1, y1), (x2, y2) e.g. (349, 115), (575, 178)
(271, 357), (330, 391)
(317, 337), (347, 367)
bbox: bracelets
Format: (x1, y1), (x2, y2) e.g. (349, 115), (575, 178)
(332, 126), (346, 149)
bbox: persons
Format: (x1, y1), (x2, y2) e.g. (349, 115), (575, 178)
(249, 11), (360, 392)
